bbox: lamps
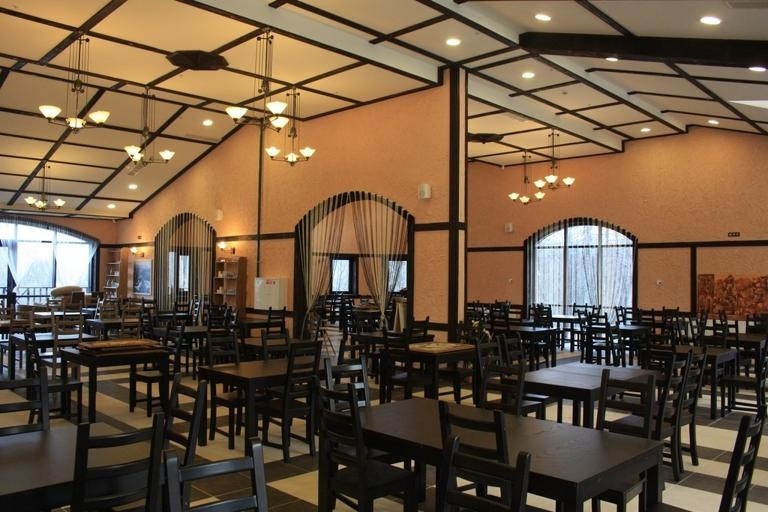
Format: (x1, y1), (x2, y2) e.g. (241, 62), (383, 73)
(22, 158), (66, 213)
(506, 149), (544, 207)
(38, 34), (111, 134)
(264, 89), (316, 168)
(227, 27), (294, 135)
(530, 127), (577, 192)
(217, 238), (233, 255)
(129, 245), (146, 258)
(117, 83), (175, 178)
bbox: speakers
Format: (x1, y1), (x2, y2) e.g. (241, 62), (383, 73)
(505, 224), (513, 233)
(418, 184), (431, 199)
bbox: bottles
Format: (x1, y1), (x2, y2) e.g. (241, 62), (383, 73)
(110, 267), (113, 274)
(106, 278), (113, 286)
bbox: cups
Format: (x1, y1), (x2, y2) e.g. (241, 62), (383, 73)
(106, 291), (116, 299)
(217, 286), (223, 294)
(114, 272), (119, 275)
(217, 270), (234, 277)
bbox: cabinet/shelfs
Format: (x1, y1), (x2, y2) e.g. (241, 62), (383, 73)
(210, 254), (249, 318)
(101, 246), (126, 297)
